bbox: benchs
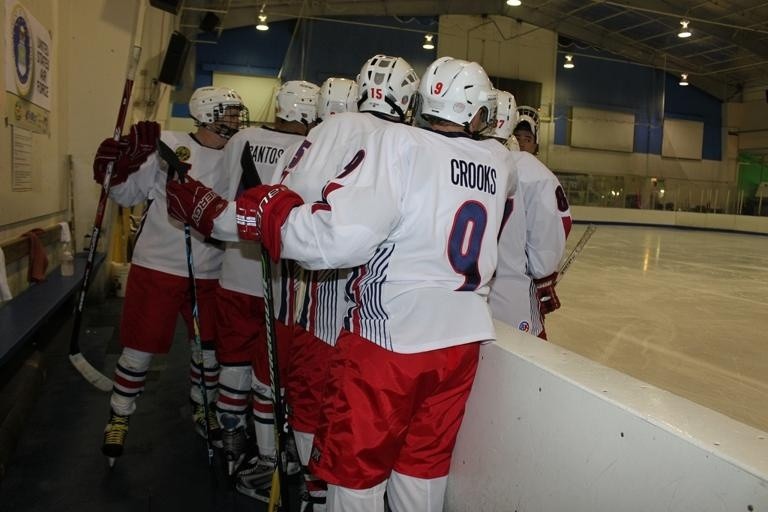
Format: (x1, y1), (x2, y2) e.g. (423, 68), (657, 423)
(0, 222), (106, 386)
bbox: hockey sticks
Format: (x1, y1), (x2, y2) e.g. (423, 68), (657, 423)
(154, 136), (216, 470)
(68, 45), (141, 391)
(240, 140), (294, 512)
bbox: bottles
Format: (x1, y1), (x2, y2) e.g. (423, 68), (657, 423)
(61, 245), (75, 276)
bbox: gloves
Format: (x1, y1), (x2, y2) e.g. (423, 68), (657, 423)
(166, 175), (229, 237)
(94, 120), (161, 185)
(236, 184), (304, 262)
(536, 284), (560, 314)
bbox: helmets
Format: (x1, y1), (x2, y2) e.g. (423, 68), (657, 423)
(518, 106), (539, 154)
(317, 77), (356, 120)
(482, 89), (520, 142)
(356, 55), (420, 127)
(276, 81), (319, 123)
(420, 56), (497, 136)
(190, 87), (250, 139)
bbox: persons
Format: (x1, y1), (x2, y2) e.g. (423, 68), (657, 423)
(492, 89), (572, 341)
(94, 77), (358, 505)
(513, 106), (539, 155)
(235, 55), (517, 511)
(173, 56), (420, 512)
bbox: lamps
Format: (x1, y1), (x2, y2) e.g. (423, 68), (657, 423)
(679, 73), (689, 86)
(564, 53), (575, 69)
(423, 32), (434, 50)
(255, 9), (269, 32)
(678, 19), (692, 38)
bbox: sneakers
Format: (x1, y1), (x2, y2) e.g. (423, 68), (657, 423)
(103, 408), (129, 456)
(192, 404), (282, 496)
